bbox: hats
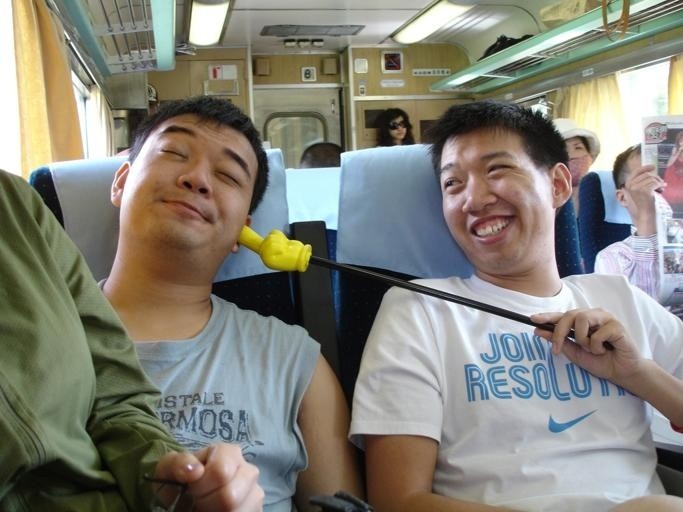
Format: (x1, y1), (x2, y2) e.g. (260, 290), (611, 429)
(552, 118), (602, 164)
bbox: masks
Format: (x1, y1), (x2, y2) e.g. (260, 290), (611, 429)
(567, 154), (594, 186)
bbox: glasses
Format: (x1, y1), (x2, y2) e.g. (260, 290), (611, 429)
(139, 472), (188, 512)
(389, 121), (407, 131)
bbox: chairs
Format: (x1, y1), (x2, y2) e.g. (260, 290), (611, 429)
(579, 173), (634, 276)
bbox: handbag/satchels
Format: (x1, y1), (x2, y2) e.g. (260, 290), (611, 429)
(540, 1), (601, 30)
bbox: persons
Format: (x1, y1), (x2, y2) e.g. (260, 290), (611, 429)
(345, 97), (683, 512)
(592, 142), (667, 303)
(80, 93), (364, 512)
(551, 117), (601, 274)
(0, 168), (269, 512)
(372, 106), (418, 146)
(298, 141), (344, 170)
